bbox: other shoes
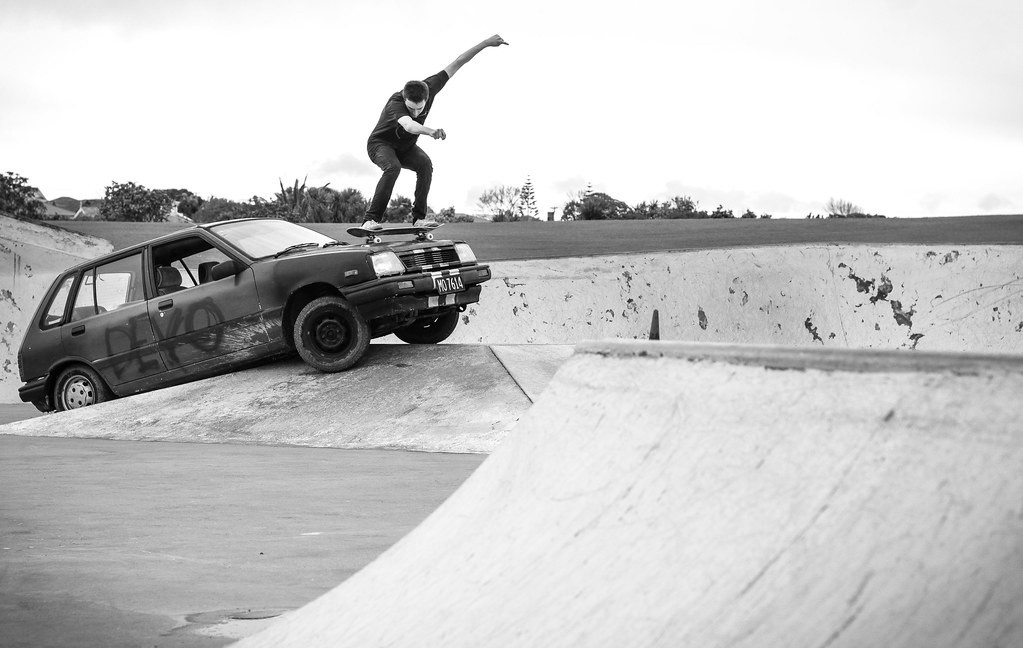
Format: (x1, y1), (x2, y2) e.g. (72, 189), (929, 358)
(414, 219), (439, 227)
(362, 220), (383, 229)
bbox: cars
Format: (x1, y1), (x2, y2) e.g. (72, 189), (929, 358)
(16, 216), (491, 412)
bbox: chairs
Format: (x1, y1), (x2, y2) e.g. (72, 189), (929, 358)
(155, 267), (189, 295)
(198, 261), (220, 285)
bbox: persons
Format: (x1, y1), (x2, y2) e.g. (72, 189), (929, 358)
(361, 34), (509, 231)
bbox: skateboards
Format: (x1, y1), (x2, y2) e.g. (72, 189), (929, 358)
(347, 222), (444, 244)
